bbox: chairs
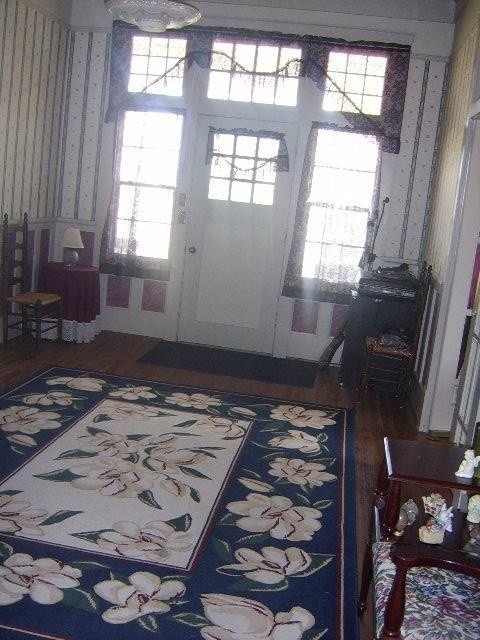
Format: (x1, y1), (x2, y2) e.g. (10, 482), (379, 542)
(361, 511), (480, 639)
(1, 212), (61, 348)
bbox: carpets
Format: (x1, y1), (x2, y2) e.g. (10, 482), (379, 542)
(136, 339), (317, 389)
(1, 365), (358, 638)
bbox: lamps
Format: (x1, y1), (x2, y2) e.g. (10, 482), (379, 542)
(62, 228), (85, 267)
(103, 1), (203, 33)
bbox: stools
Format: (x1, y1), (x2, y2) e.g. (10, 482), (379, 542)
(360, 335), (415, 411)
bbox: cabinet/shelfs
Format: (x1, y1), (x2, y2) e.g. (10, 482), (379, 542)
(339, 296), (422, 390)
